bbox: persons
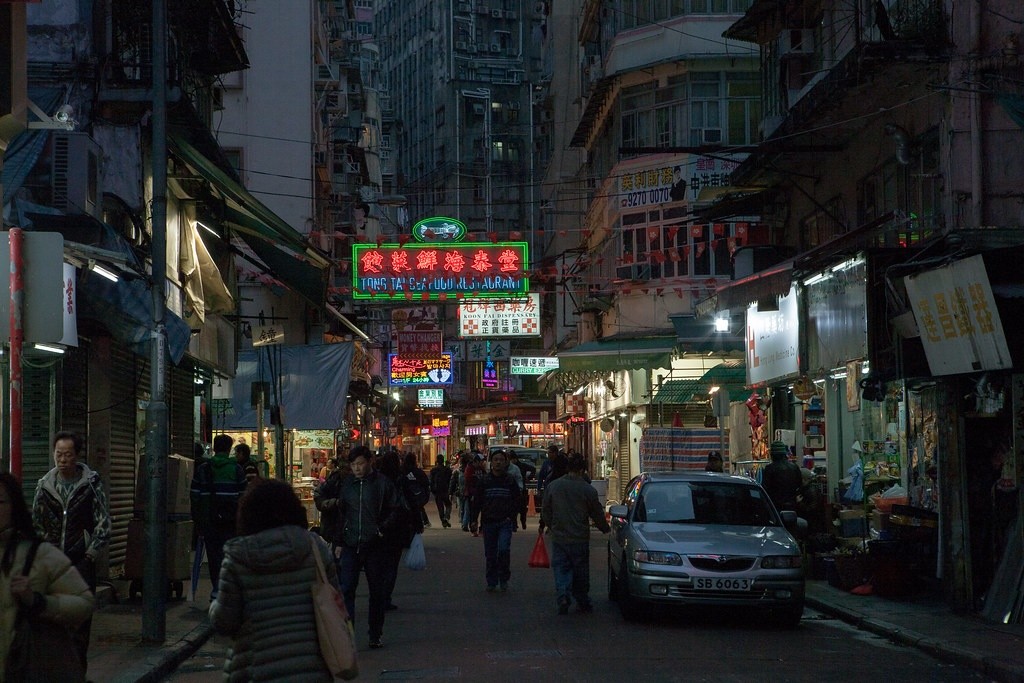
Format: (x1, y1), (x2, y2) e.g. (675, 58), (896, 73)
(706, 441), (826, 536)
(851, 554), (887, 595)
(429, 449), (536, 537)
(0, 431), (112, 683)
(537, 445), (592, 573)
(208, 477), (345, 683)
(191, 435), (260, 604)
(310, 442), (429, 649)
(469, 450), (521, 593)
(542, 456), (611, 615)
(925, 447), (938, 484)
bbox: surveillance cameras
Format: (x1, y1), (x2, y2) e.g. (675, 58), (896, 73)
(55, 104), (73, 123)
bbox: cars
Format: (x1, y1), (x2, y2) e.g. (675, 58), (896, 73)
(607, 469), (804, 628)
(485, 444), (549, 481)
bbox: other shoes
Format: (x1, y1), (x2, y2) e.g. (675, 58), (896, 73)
(559, 600), (569, 615)
(369, 639), (384, 648)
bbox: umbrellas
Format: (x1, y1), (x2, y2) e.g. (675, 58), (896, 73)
(192, 534), (205, 602)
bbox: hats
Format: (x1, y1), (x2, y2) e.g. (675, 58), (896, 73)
(708, 452), (722, 461)
(770, 441), (786, 454)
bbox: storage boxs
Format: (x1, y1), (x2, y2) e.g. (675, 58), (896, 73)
(134, 453), (195, 521)
(839, 509), (868, 538)
(125, 517), (193, 580)
(823, 557), (850, 589)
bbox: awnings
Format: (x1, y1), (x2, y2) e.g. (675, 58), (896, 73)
(669, 310), (745, 353)
(167, 131), (331, 314)
(27, 202), (190, 364)
(556, 338), (674, 373)
(895, 226), (1024, 279)
(721, 0), (826, 44)
(714, 261), (794, 313)
(652, 362), (767, 403)
(220, 342), (355, 433)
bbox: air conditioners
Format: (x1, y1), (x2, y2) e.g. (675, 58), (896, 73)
(506, 11), (516, 19)
(492, 9), (502, 18)
(459, 3), (471, 12)
(491, 44), (500, 52)
(315, 62), (341, 86)
(317, 92), (347, 114)
(346, 162), (360, 173)
(465, 61), (478, 68)
(478, 43), (488, 51)
(456, 41), (466, 49)
(700, 128), (722, 145)
(478, 6), (489, 13)
(507, 48), (517, 56)
(52, 132), (103, 219)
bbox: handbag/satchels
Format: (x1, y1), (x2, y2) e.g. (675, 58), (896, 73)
(404, 532), (426, 570)
(528, 535), (549, 568)
(311, 536), (359, 681)
(5, 540), (84, 683)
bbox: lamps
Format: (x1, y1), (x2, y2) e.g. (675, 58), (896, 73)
(88, 258), (121, 283)
(31, 342), (67, 354)
(27, 96), (79, 131)
(325, 195), (408, 208)
